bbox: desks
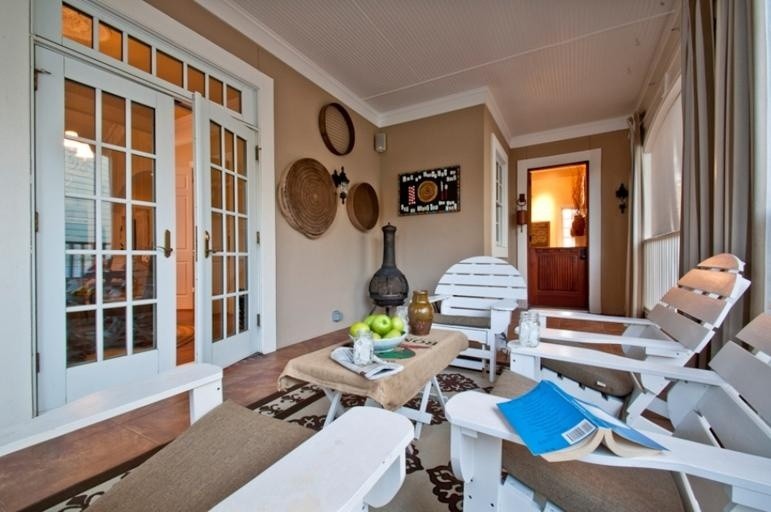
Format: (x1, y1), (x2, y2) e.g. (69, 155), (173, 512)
(275, 324), (468, 451)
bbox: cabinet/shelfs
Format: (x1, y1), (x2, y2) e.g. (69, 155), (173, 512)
(364, 297), (404, 320)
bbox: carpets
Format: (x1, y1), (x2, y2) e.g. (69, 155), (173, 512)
(45, 358), (512, 512)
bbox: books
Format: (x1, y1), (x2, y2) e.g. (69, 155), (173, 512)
(495, 380), (671, 463)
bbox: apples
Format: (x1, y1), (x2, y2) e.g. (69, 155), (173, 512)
(350, 314), (404, 339)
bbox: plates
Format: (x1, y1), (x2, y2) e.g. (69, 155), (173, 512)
(347, 329), (407, 352)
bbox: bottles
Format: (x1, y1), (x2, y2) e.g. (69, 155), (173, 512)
(518, 312), (540, 346)
(353, 330), (374, 365)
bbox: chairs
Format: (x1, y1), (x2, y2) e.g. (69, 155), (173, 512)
(515, 253), (750, 419)
(443, 313), (769, 512)
(407, 257), (528, 384)
(1, 355), (416, 511)
